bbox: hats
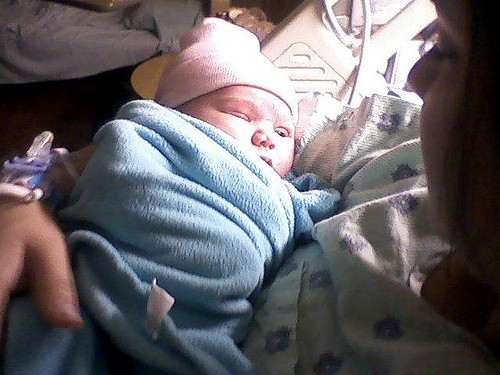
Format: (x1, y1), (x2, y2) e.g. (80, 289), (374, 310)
(155, 17), (299, 126)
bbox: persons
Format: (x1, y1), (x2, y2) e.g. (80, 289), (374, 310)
(5, 17), (344, 375)
(1, 0), (500, 375)
